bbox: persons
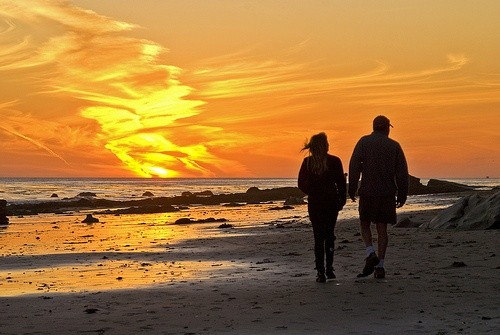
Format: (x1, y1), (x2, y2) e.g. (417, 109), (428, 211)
(298, 131), (347, 283)
(347, 115), (409, 279)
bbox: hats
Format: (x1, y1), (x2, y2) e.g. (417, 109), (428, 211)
(374, 115), (393, 127)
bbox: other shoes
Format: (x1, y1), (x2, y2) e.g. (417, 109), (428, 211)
(357, 255), (381, 278)
(326, 270), (336, 279)
(373, 268), (384, 278)
(316, 277), (326, 283)
